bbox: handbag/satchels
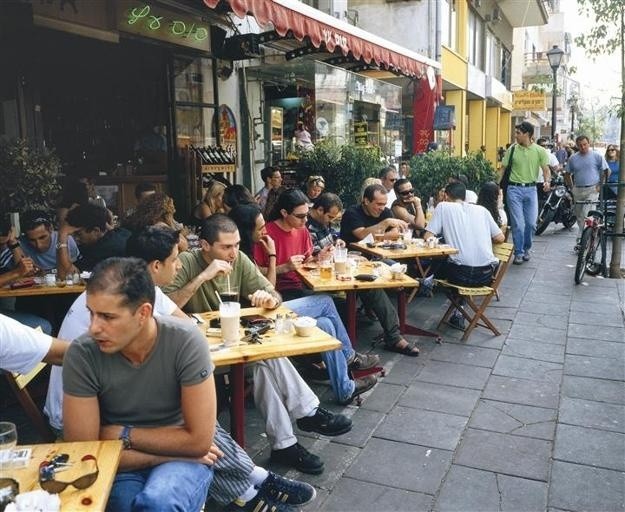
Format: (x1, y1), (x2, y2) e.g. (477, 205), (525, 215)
(501, 161), (511, 189)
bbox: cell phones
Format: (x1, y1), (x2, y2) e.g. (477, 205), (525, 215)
(354, 274), (378, 282)
(11, 277), (35, 289)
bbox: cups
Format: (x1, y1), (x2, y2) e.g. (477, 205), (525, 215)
(348, 251), (361, 270)
(403, 229), (413, 245)
(294, 316), (319, 337)
(0, 421), (16, 473)
(425, 210), (433, 220)
(374, 228), (385, 247)
(216, 282), (241, 304)
(332, 247), (347, 274)
(218, 300), (240, 349)
(318, 252), (333, 280)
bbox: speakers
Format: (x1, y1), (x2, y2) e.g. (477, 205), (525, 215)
(210, 24), (227, 56)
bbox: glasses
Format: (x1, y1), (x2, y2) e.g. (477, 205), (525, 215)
(397, 189), (413, 196)
(38, 455), (99, 493)
(291, 208), (310, 218)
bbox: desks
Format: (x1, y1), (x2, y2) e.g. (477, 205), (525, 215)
(294, 260), (419, 408)
(349, 239), (459, 348)
(186, 303), (343, 449)
(0, 278), (86, 317)
(0, 439), (123, 511)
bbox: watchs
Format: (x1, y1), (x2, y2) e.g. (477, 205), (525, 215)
(117, 423), (134, 451)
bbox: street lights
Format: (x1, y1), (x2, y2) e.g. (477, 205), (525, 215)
(567, 93), (580, 143)
(547, 44), (565, 144)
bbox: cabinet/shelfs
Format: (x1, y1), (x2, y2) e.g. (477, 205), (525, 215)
(187, 147), (237, 218)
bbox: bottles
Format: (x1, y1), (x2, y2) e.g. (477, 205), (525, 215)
(65, 268), (79, 287)
(197, 172), (230, 187)
(183, 142), (234, 164)
(428, 196), (434, 210)
(274, 310), (293, 336)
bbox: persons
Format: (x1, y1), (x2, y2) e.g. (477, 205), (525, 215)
(161, 210), (354, 476)
(501, 121), (551, 263)
(59, 254), (223, 512)
(294, 121), (311, 148)
(1, 162), (507, 405)
(39, 222), (318, 512)
(535, 135), (620, 248)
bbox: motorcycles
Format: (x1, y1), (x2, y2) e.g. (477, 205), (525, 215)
(534, 163), (580, 238)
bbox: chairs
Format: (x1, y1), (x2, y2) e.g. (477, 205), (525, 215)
(6, 326), (56, 444)
(433, 240), (515, 341)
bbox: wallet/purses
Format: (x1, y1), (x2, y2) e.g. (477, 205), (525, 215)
(354, 275), (376, 281)
(240, 315), (269, 326)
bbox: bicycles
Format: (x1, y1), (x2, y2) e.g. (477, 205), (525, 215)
(572, 198), (619, 285)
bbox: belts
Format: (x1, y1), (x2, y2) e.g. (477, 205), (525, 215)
(506, 182), (536, 187)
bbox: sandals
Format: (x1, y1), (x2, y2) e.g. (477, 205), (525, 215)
(387, 339), (419, 355)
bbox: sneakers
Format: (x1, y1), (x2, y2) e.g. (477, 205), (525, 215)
(229, 353), (379, 511)
(512, 255), (530, 264)
(450, 316), (466, 329)
